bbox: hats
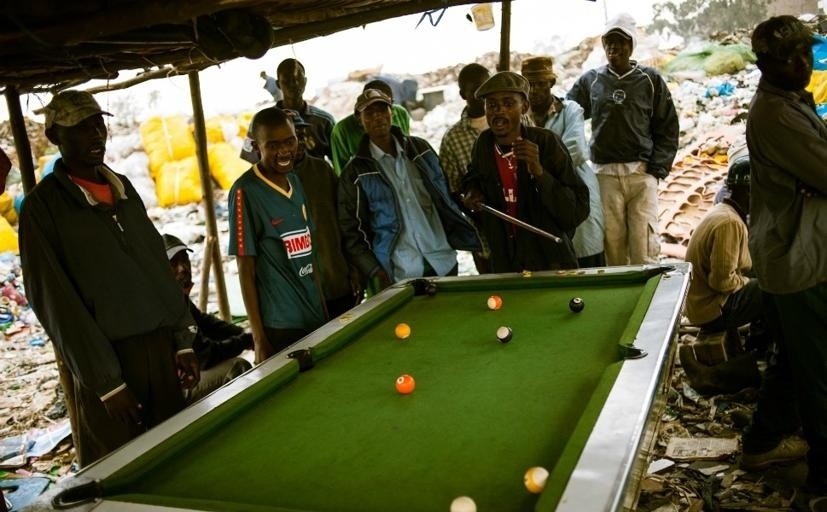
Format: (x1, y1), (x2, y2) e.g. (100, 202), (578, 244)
(521, 57), (558, 82)
(475, 72), (530, 101)
(356, 89), (392, 112)
(752, 15), (813, 52)
(45, 90), (113, 130)
(162, 234), (193, 260)
(283, 109), (314, 128)
(601, 26), (638, 55)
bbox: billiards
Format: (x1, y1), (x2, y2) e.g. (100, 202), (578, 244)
(496, 326), (512, 343)
(523, 466), (549, 493)
(394, 323), (411, 339)
(426, 284), (437, 297)
(395, 374), (415, 395)
(486, 294), (502, 310)
(568, 297), (584, 313)
(449, 495), (476, 512)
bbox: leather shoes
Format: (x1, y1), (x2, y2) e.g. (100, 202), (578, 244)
(740, 438), (809, 472)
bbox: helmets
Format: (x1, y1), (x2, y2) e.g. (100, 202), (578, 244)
(725, 159), (750, 186)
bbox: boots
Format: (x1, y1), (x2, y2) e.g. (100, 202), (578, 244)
(680, 345), (759, 396)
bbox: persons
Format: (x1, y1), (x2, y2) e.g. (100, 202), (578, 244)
(686, 160), (776, 359)
(228, 108), (364, 367)
(337, 90), (481, 295)
(519, 57), (606, 269)
(162, 234), (254, 407)
(332, 80), (409, 176)
(19, 91), (199, 470)
(739, 15), (825, 491)
(567, 26), (678, 266)
(241, 59), (333, 167)
(440, 65), (503, 274)
(458, 71), (591, 272)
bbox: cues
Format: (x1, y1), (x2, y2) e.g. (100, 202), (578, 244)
(460, 193), (561, 244)
